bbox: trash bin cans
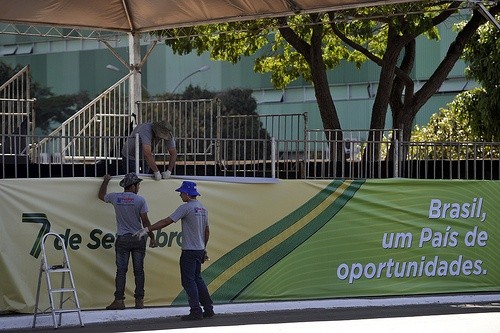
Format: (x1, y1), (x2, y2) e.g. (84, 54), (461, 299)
(210, 144), (234, 177)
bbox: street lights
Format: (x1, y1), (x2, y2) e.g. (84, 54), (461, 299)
(106, 65), (151, 97)
(171, 66), (211, 96)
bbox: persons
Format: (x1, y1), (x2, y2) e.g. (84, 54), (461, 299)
(122, 120), (176, 180)
(97, 174), (158, 310)
(131, 181), (216, 321)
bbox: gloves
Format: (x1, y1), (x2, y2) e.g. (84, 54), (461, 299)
(161, 170), (171, 178)
(132, 227), (149, 240)
(155, 171), (162, 179)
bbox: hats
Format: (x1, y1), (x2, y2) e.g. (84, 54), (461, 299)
(175, 181), (200, 195)
(152, 120), (174, 140)
(120, 172), (142, 187)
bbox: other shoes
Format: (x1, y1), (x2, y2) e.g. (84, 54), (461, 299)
(135, 298), (144, 308)
(182, 312), (203, 320)
(202, 311), (215, 317)
(106, 299), (125, 310)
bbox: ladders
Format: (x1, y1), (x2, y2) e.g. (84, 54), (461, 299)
(32, 233), (85, 330)
